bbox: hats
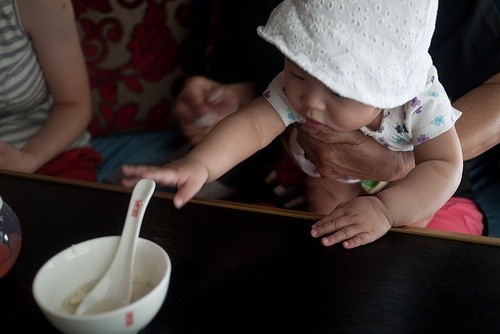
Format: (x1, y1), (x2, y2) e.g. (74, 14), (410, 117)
(253, 0), (441, 104)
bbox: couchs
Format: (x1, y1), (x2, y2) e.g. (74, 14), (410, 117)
(70, 0), (244, 200)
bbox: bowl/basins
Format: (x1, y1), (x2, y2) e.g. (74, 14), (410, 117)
(31, 235), (172, 334)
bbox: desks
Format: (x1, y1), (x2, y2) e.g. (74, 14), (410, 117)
(0, 169), (500, 334)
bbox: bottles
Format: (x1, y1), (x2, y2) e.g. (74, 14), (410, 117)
(0, 195), (23, 278)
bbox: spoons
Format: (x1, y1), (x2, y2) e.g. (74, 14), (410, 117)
(74, 178), (157, 316)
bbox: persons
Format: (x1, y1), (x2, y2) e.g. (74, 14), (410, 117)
(162, 0), (500, 238)
(0, 0), (167, 191)
(121, 0), (463, 249)
